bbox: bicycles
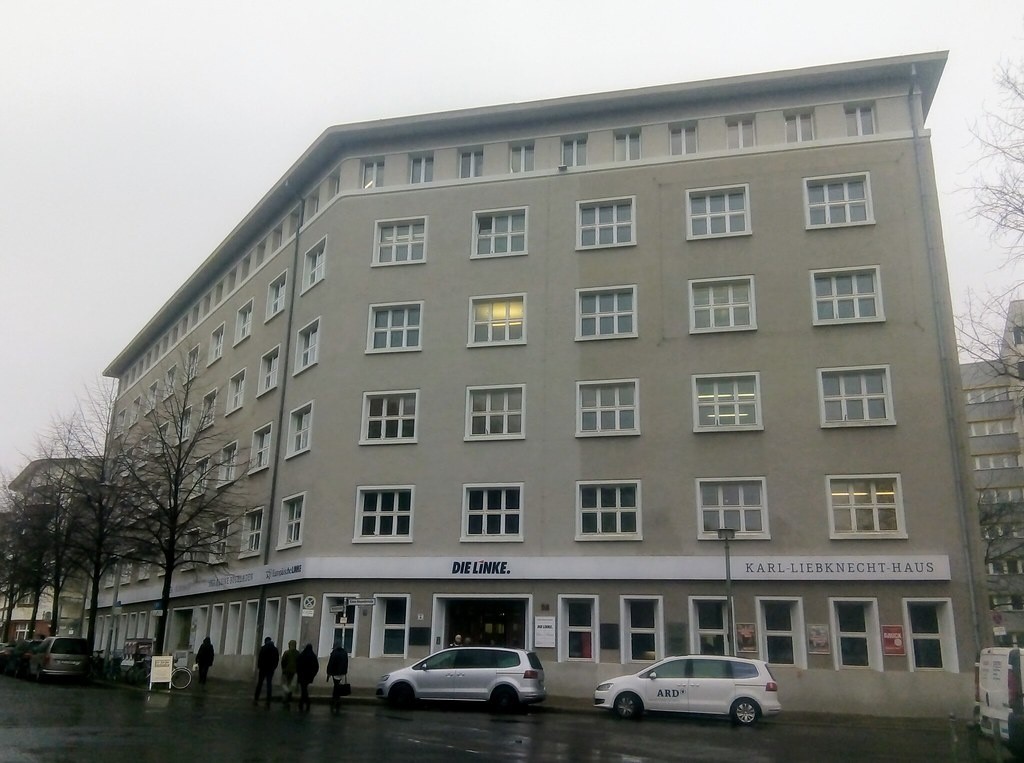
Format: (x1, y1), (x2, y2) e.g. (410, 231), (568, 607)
(136, 658), (194, 691)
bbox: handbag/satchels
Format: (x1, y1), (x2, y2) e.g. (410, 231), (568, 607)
(338, 683), (352, 696)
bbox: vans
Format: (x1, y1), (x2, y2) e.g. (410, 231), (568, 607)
(978, 646), (1024, 740)
(29, 637), (90, 686)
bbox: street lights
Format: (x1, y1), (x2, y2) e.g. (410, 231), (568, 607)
(718, 528), (735, 657)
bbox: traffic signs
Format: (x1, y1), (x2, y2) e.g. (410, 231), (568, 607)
(330, 605), (344, 613)
(348, 597), (376, 606)
(340, 617), (347, 623)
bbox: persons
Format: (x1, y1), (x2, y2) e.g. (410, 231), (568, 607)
(450, 632), (499, 647)
(252, 636), (280, 703)
(297, 643), (319, 711)
(280, 640), (302, 704)
(195, 636), (214, 685)
(325, 639), (349, 712)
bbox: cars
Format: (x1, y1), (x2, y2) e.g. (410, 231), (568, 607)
(376, 645), (547, 714)
(591, 654), (783, 725)
(1, 639), (44, 682)
